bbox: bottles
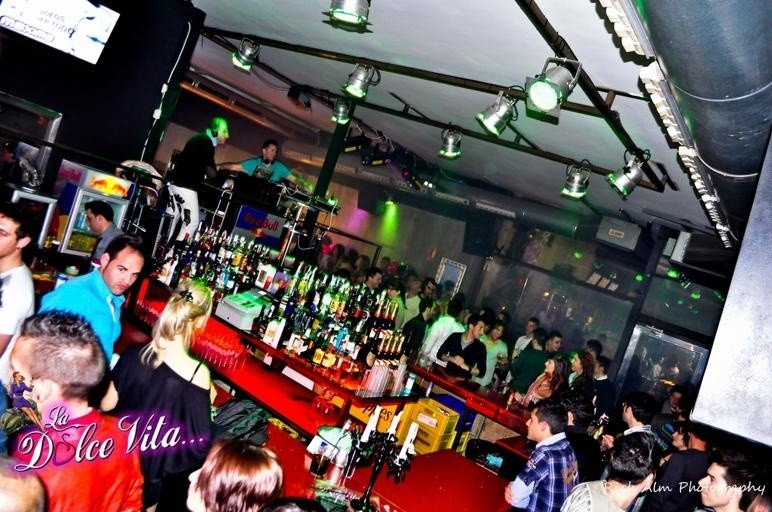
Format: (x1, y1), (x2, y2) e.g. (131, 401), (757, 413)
(156, 221), (294, 306)
(256, 261), (416, 394)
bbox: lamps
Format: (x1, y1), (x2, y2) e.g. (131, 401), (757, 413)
(332, 97), (351, 124)
(329, 0), (369, 26)
(561, 164), (591, 198)
(288, 88), (312, 112)
(475, 89), (511, 137)
(526, 57), (581, 119)
(401, 170), (423, 192)
(606, 155), (643, 196)
(342, 63), (374, 97)
(361, 154), (390, 166)
(440, 129), (464, 157)
(342, 137), (372, 153)
(234, 37), (260, 71)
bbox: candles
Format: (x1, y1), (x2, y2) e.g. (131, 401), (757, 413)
(372, 405), (381, 431)
(387, 410), (403, 441)
(360, 411), (374, 444)
(396, 420), (419, 466)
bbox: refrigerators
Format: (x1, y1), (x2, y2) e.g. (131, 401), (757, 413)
(48, 157), (133, 260)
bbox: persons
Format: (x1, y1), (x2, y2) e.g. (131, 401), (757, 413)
(0, 114), (771, 512)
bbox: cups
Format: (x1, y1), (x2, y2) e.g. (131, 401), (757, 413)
(308, 446), (334, 480)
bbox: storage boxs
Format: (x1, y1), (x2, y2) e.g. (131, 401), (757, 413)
(412, 427), (451, 455)
(410, 398), (460, 434)
(446, 432), (471, 453)
(429, 393), (475, 432)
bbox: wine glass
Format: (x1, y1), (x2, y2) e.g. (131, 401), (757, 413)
(495, 350), (506, 368)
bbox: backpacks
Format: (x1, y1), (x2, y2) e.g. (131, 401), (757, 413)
(213, 399), (270, 447)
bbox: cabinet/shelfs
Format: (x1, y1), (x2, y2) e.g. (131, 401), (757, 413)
(122, 269), (417, 442)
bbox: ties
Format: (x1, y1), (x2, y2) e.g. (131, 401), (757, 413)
(90, 237), (103, 261)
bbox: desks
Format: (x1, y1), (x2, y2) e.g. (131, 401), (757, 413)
(496, 435), (534, 477)
(279, 447), (511, 511)
(413, 353), (529, 435)
